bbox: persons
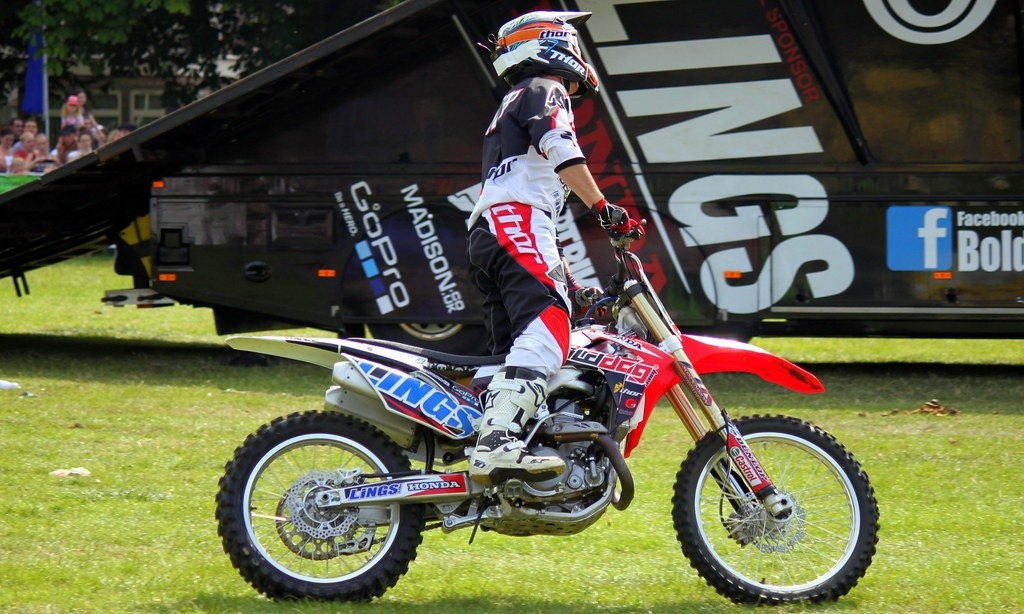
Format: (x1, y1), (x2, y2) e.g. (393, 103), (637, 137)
(467, 11), (647, 490)
(0, 90), (137, 179)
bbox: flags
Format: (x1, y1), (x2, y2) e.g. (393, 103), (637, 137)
(20, 29), (44, 116)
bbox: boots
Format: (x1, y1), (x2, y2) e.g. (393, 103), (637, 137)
(469, 365), (566, 485)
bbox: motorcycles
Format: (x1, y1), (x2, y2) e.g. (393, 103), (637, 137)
(216, 212), (883, 608)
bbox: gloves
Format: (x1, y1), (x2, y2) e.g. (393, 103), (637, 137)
(590, 198), (630, 233)
(566, 273), (590, 317)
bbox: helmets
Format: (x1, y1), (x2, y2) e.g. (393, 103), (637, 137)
(477, 10), (600, 111)
